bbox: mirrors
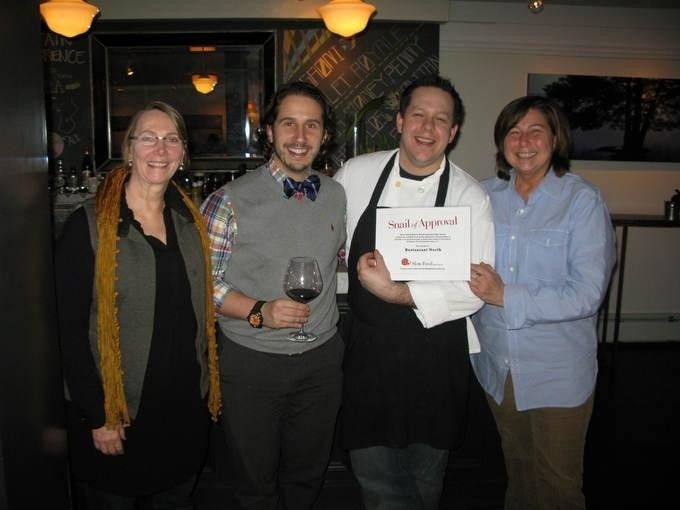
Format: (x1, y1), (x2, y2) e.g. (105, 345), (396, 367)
(84, 27), (282, 178)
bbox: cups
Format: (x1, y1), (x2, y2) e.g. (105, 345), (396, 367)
(663, 200), (678, 221)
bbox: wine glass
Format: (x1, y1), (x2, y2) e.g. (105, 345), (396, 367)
(283, 255), (323, 342)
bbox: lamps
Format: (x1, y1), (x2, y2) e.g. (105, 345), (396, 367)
(186, 48), (222, 97)
(526, 0), (545, 14)
(39, 0), (100, 38)
(317, 0), (376, 38)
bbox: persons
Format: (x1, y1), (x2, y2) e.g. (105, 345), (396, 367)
(465, 96), (618, 510)
(193, 77), (347, 507)
(56, 99), (223, 510)
(331, 75), (496, 510)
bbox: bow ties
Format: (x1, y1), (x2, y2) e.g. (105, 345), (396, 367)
(283, 175), (321, 201)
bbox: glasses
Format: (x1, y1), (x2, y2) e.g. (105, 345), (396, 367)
(126, 133), (184, 147)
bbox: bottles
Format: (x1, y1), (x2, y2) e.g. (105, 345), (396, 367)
(51, 149), (243, 206)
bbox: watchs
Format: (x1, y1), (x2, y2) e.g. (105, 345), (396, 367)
(247, 300), (268, 329)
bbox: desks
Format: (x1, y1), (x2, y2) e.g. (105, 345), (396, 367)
(590, 214), (679, 358)
